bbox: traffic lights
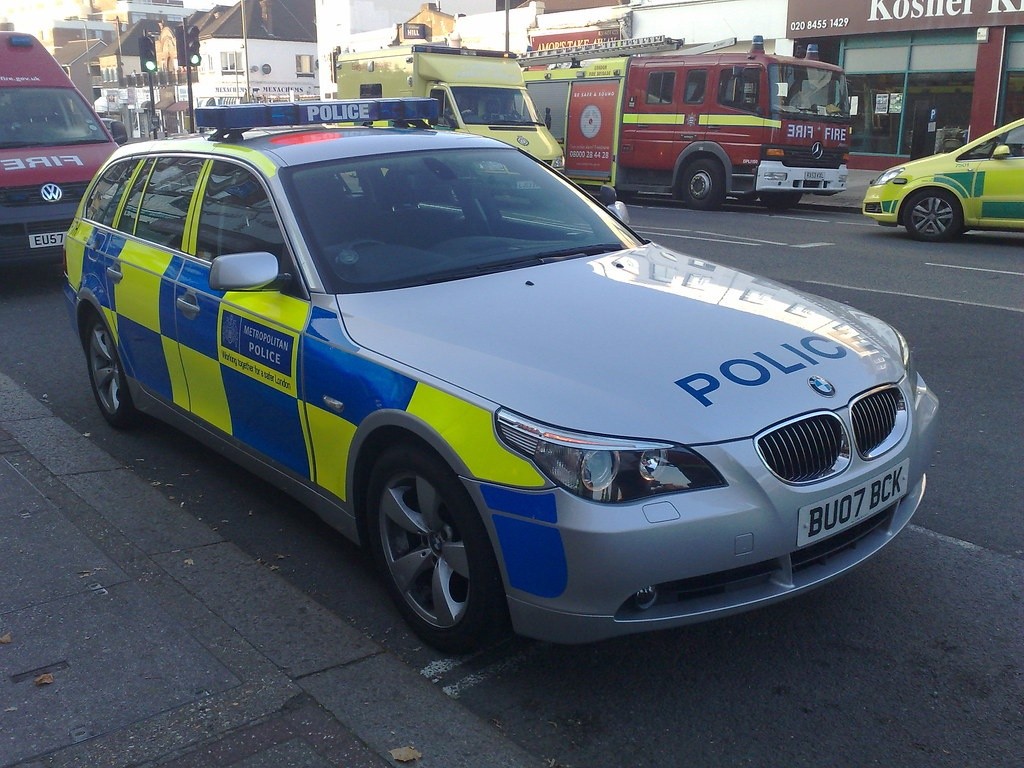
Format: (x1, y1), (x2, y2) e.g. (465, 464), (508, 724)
(138, 35), (158, 72)
(184, 25), (200, 67)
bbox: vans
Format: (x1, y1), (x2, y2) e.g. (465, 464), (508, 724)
(1, 26), (132, 296)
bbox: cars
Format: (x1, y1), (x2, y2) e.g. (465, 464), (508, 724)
(862, 116), (1024, 239)
(58, 89), (940, 661)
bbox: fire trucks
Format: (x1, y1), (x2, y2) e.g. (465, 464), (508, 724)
(520, 27), (852, 209)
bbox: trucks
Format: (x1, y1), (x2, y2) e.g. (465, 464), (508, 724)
(334, 36), (567, 208)
